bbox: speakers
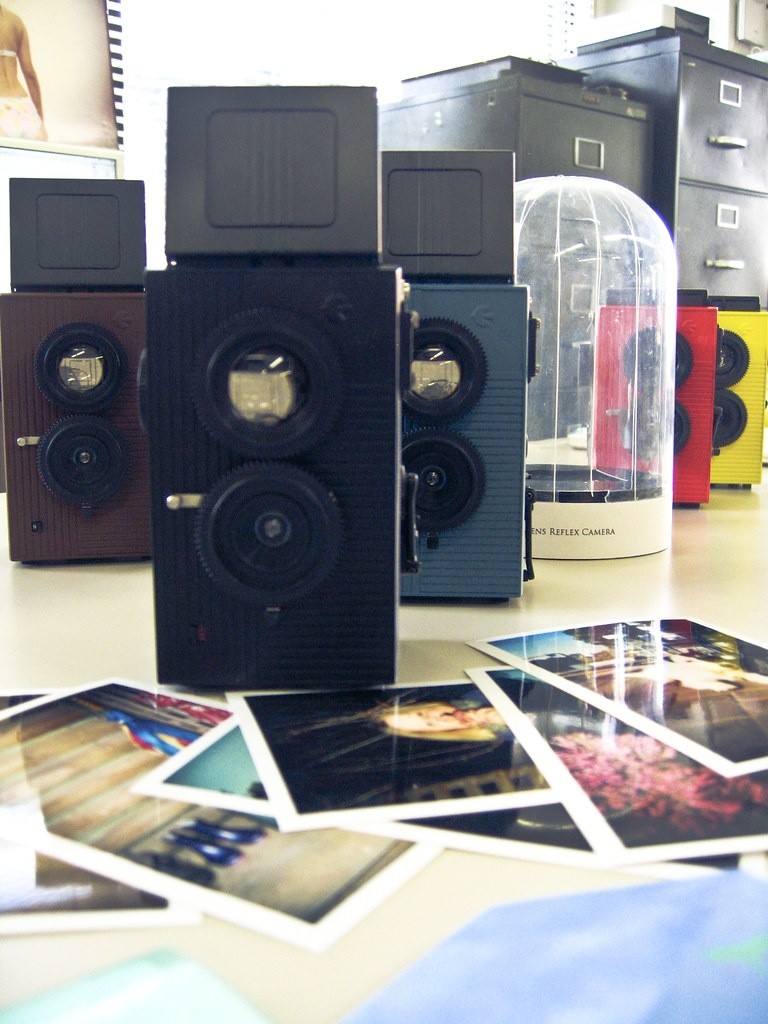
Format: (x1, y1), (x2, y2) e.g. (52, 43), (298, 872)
(0, 261), (541, 691)
(590, 306), (768, 503)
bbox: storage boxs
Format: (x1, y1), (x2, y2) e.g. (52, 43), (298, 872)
(378, 55), (655, 181)
(578, 25), (768, 308)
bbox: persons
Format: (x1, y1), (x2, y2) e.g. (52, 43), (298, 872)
(0, 2), (50, 143)
